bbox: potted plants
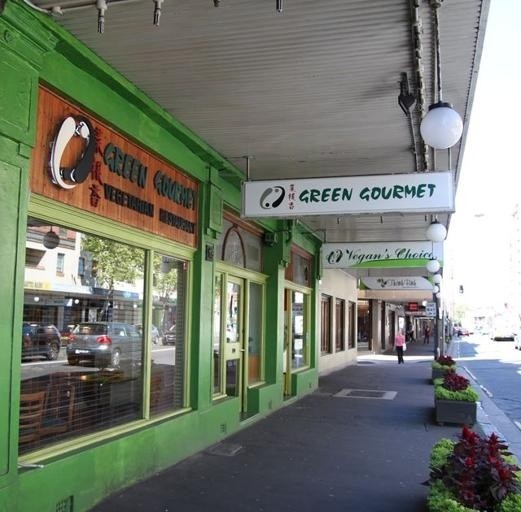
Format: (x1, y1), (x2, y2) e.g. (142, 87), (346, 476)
(421, 350), (520, 512)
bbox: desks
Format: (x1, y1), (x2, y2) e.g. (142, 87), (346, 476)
(65, 374), (137, 429)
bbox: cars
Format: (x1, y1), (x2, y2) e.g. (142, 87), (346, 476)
(449, 320), (521, 352)
(136, 322), (160, 344)
(22, 322), (62, 360)
(163, 323), (177, 344)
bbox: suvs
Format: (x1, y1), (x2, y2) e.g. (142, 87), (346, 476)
(67, 321), (143, 366)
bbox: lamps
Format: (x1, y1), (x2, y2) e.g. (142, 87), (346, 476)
(420, 0), (465, 149)
(425, 213), (448, 243)
(425, 241), (441, 273)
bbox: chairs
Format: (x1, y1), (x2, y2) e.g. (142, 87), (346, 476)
(43, 382), (76, 435)
(150, 375), (161, 416)
(20, 390), (49, 445)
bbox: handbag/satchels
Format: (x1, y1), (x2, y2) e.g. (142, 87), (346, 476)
(402, 343), (407, 351)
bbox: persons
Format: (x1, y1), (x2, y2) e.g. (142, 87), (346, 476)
(407, 323), (417, 343)
(393, 329), (406, 365)
(423, 325), (431, 344)
